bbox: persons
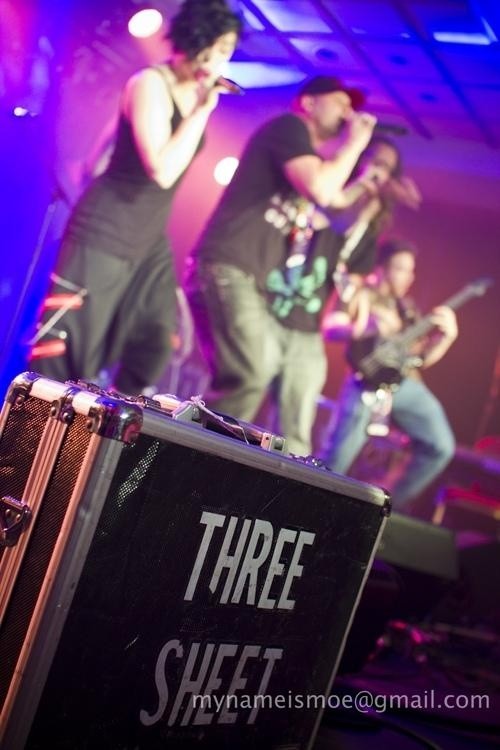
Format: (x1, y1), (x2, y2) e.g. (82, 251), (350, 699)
(186, 73), (379, 426)
(32, 2), (247, 384)
(262, 133), (406, 462)
(321, 234), (461, 510)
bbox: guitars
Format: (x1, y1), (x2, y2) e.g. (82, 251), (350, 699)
(344, 277), (495, 391)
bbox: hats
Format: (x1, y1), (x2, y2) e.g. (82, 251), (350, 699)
(300, 75), (366, 113)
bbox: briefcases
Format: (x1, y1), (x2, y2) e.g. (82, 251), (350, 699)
(0, 371), (393, 750)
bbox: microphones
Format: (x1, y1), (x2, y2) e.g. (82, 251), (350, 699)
(371, 174), (388, 210)
(202, 69), (244, 97)
(341, 116), (409, 136)
